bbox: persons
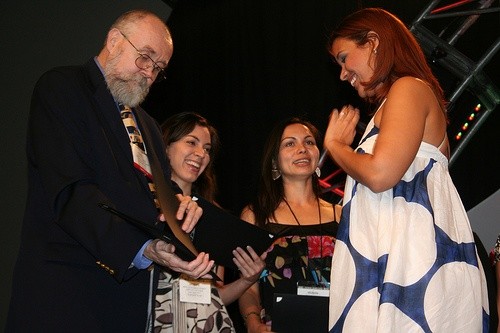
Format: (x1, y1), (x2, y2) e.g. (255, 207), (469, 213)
(145, 110), (266, 333)
(236, 116), (343, 333)
(7, 9), (216, 333)
(323, 7), (492, 333)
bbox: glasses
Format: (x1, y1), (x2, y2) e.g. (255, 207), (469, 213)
(119, 31), (167, 82)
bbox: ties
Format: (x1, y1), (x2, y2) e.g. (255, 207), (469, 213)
(118, 102), (147, 154)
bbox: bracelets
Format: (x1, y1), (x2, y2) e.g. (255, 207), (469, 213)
(244, 312), (260, 321)
